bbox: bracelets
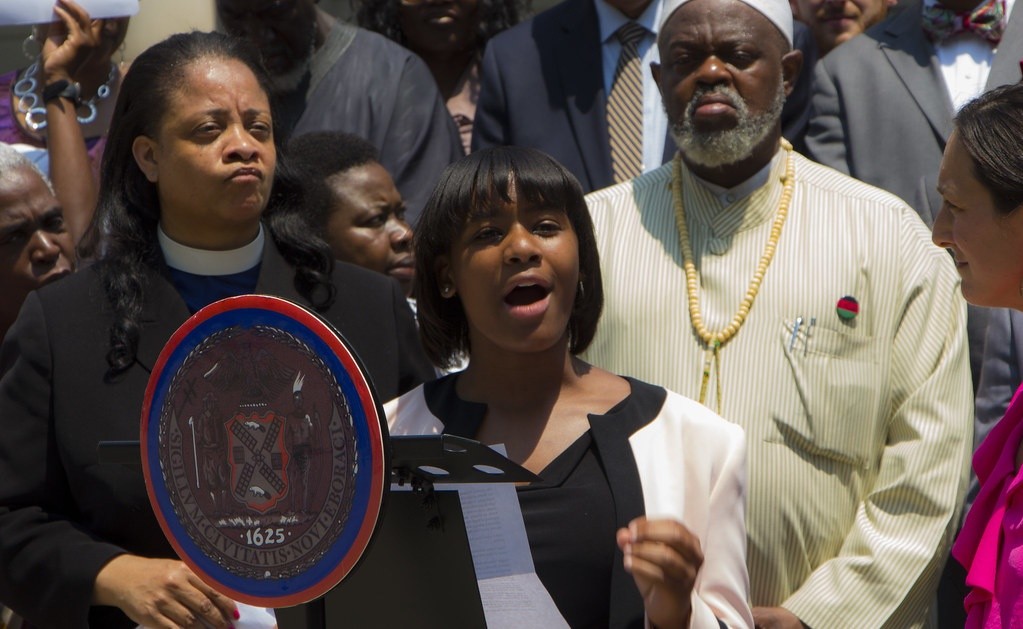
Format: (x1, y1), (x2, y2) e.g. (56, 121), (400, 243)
(44, 81), (81, 104)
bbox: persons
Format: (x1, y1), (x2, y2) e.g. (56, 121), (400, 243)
(0, 0), (1023, 629)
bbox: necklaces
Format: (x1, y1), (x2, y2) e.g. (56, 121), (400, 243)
(14, 57), (116, 132)
(673, 137), (794, 413)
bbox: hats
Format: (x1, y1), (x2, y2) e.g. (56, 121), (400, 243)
(657, 0), (794, 51)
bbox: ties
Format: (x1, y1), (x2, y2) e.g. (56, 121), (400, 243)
(606, 21), (646, 184)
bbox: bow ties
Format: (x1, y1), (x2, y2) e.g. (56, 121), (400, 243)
(921, 0), (1008, 51)
